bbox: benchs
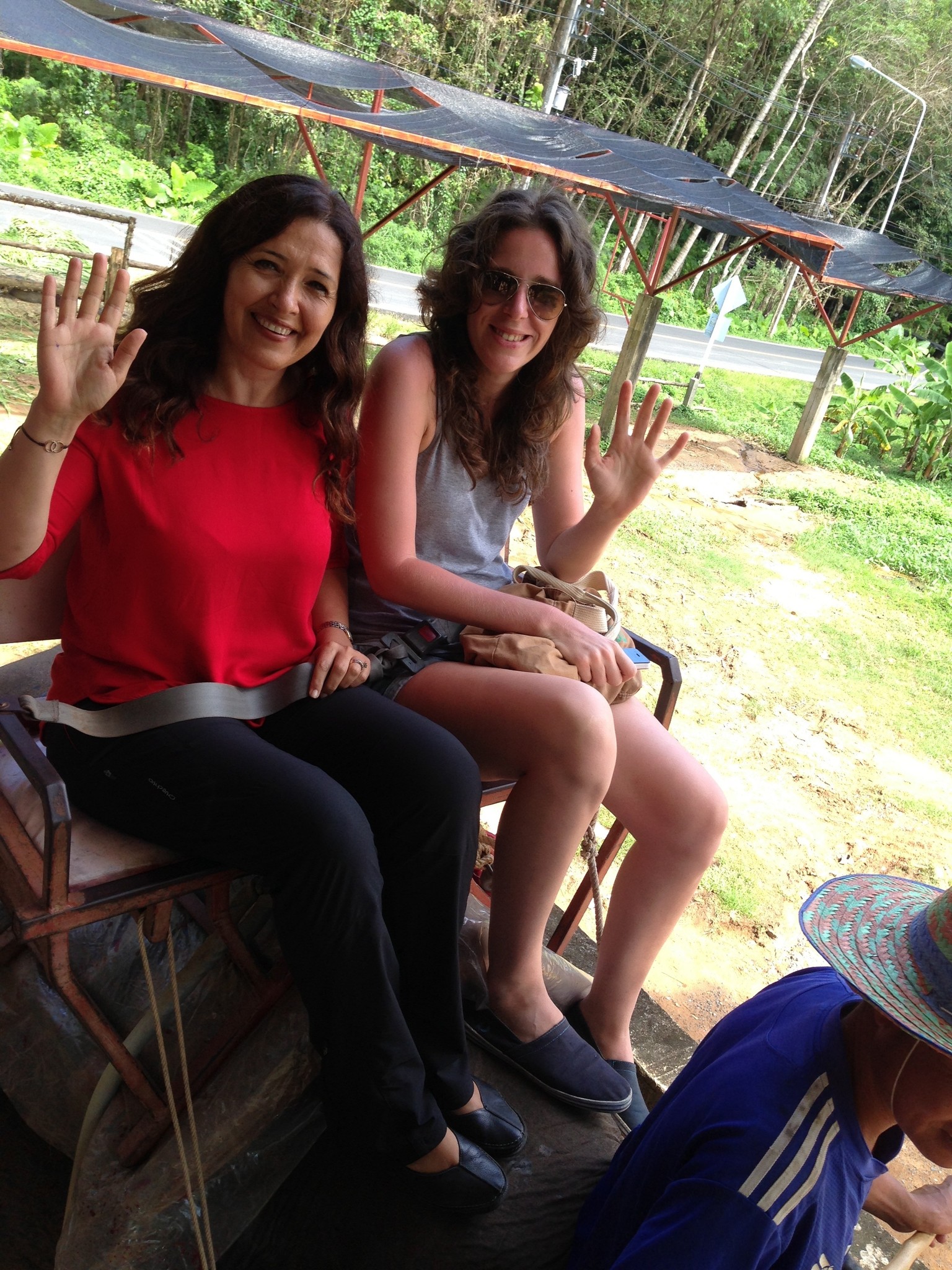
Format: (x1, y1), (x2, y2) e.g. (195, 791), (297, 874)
(0, 616), (684, 1176)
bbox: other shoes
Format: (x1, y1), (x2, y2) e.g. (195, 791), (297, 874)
(400, 1135), (510, 1215)
(442, 1080), (529, 1160)
(463, 992), (633, 1115)
(565, 1000), (651, 1138)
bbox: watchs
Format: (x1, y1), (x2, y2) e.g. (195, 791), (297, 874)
(315, 620), (353, 645)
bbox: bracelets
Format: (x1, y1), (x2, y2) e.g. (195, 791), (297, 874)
(8, 425), (73, 452)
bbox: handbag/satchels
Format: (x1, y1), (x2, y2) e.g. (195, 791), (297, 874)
(459, 562), (643, 705)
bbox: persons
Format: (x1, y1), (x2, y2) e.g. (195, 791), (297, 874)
(570, 874), (952, 1270)
(0, 174), (526, 1212)
(338, 191), (729, 1134)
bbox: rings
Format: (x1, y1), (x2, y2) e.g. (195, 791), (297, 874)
(350, 659), (368, 673)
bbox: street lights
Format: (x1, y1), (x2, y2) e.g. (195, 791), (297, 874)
(846, 43), (927, 340)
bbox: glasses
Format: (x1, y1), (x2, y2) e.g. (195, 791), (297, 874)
(475, 266), (569, 321)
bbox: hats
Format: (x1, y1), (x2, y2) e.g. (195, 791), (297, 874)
(800, 873), (952, 1059)
